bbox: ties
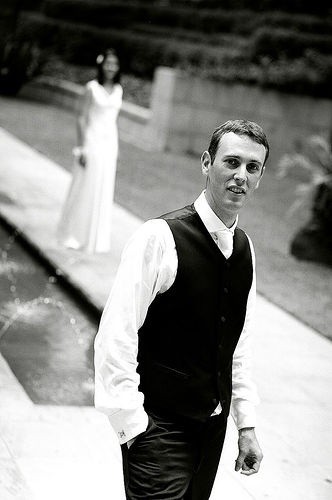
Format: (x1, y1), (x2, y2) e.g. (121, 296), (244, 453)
(215, 229), (234, 259)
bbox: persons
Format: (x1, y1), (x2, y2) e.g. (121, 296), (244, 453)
(59, 48), (123, 254)
(92, 119), (270, 500)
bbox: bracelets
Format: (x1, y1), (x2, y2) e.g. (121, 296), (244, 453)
(72, 146), (81, 156)
